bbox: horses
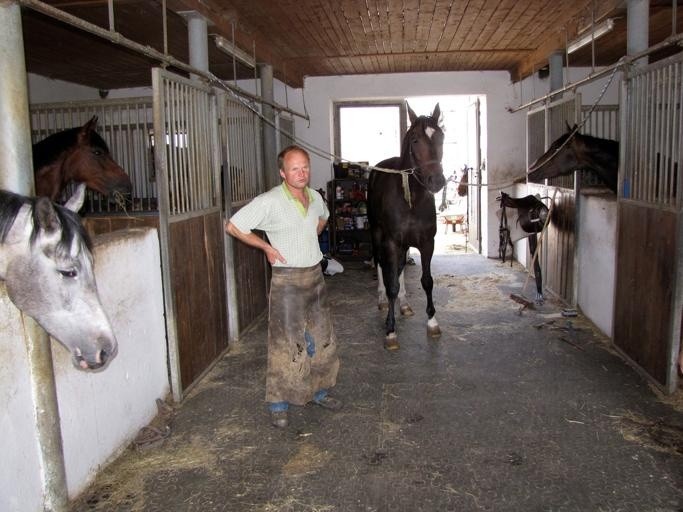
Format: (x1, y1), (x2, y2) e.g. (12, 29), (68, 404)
(31, 114), (134, 207)
(528, 119), (678, 201)
(366, 100), (447, 352)
(0, 183), (119, 374)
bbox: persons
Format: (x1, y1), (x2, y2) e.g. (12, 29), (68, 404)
(225, 146), (343, 427)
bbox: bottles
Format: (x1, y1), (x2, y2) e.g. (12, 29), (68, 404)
(348, 181), (367, 200)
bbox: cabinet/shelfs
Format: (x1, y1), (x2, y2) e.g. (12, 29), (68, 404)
(327, 177), (373, 260)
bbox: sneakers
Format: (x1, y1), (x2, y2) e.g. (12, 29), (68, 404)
(270, 408), (289, 428)
(310, 396), (343, 411)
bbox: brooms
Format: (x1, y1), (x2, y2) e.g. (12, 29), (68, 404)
(510, 187), (559, 308)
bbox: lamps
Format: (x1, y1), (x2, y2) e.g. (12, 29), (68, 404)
(215, 36), (257, 69)
(567, 18), (614, 53)
